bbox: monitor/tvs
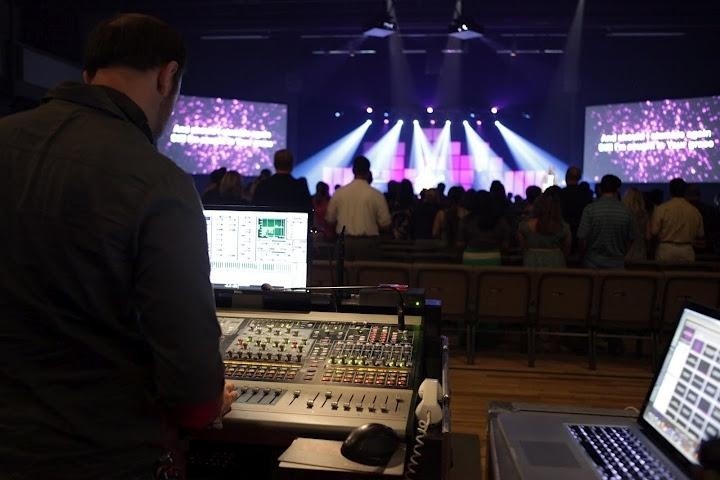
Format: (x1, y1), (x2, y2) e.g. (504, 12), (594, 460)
(199, 202), (313, 310)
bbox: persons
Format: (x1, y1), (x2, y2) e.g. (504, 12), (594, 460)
(0, 14), (237, 479)
(200, 149), (707, 268)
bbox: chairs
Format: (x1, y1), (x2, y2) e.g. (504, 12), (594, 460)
(595, 269), (665, 368)
(308, 261), (336, 295)
(344, 258), (410, 299)
(532, 267), (595, 370)
(411, 263), (470, 363)
(659, 271), (719, 365)
(471, 264), (532, 368)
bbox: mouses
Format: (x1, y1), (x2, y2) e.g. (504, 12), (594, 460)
(339, 423), (397, 467)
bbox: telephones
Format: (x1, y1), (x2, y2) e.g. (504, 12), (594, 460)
(415, 378), (443, 425)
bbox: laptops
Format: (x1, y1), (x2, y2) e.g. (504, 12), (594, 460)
(493, 300), (720, 480)
